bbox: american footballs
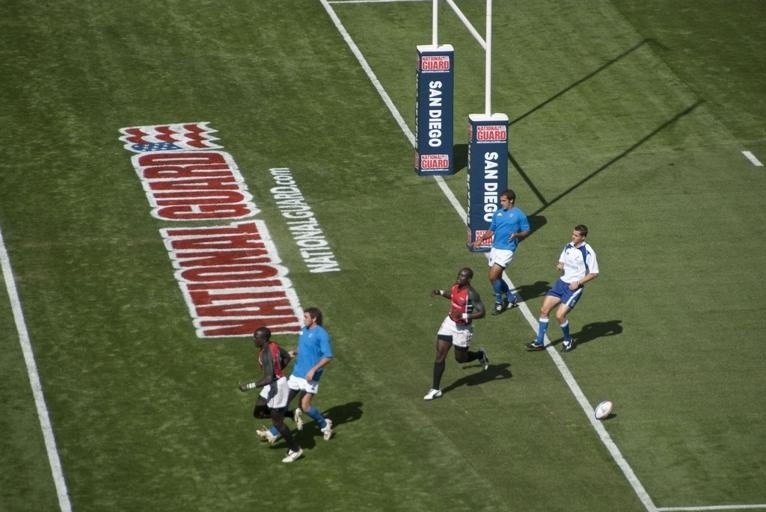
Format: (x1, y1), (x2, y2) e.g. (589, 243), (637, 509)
(595, 401), (611, 420)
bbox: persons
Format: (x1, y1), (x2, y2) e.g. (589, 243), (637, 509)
(256, 307), (333, 444)
(524, 224), (600, 352)
(476, 190), (531, 315)
(424, 268), (489, 400)
(238, 327), (304, 463)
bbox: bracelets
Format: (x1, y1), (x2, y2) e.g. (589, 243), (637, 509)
(462, 312), (467, 320)
(440, 290), (444, 296)
(247, 382), (256, 390)
(577, 280), (581, 288)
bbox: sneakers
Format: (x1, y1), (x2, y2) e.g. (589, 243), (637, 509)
(319, 418), (335, 440)
(478, 348), (489, 370)
(495, 296), (517, 314)
(256, 430), (277, 445)
(281, 447), (303, 464)
(293, 407), (305, 430)
(525, 341), (544, 351)
(561, 337), (574, 352)
(423, 388), (442, 400)
